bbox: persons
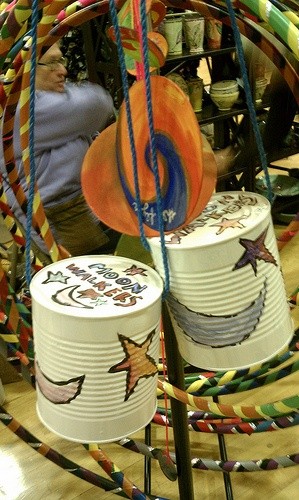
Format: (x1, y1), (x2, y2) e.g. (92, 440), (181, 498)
(28, 40), (119, 253)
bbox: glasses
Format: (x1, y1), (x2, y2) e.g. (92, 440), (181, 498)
(36, 57), (68, 70)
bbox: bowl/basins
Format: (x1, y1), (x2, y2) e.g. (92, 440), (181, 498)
(209, 80), (240, 111)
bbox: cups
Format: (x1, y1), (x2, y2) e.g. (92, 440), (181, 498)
(187, 78), (203, 113)
(207, 19), (223, 49)
(164, 18), (183, 56)
(184, 17), (206, 53)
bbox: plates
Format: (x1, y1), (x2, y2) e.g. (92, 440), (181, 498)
(255, 174), (299, 200)
